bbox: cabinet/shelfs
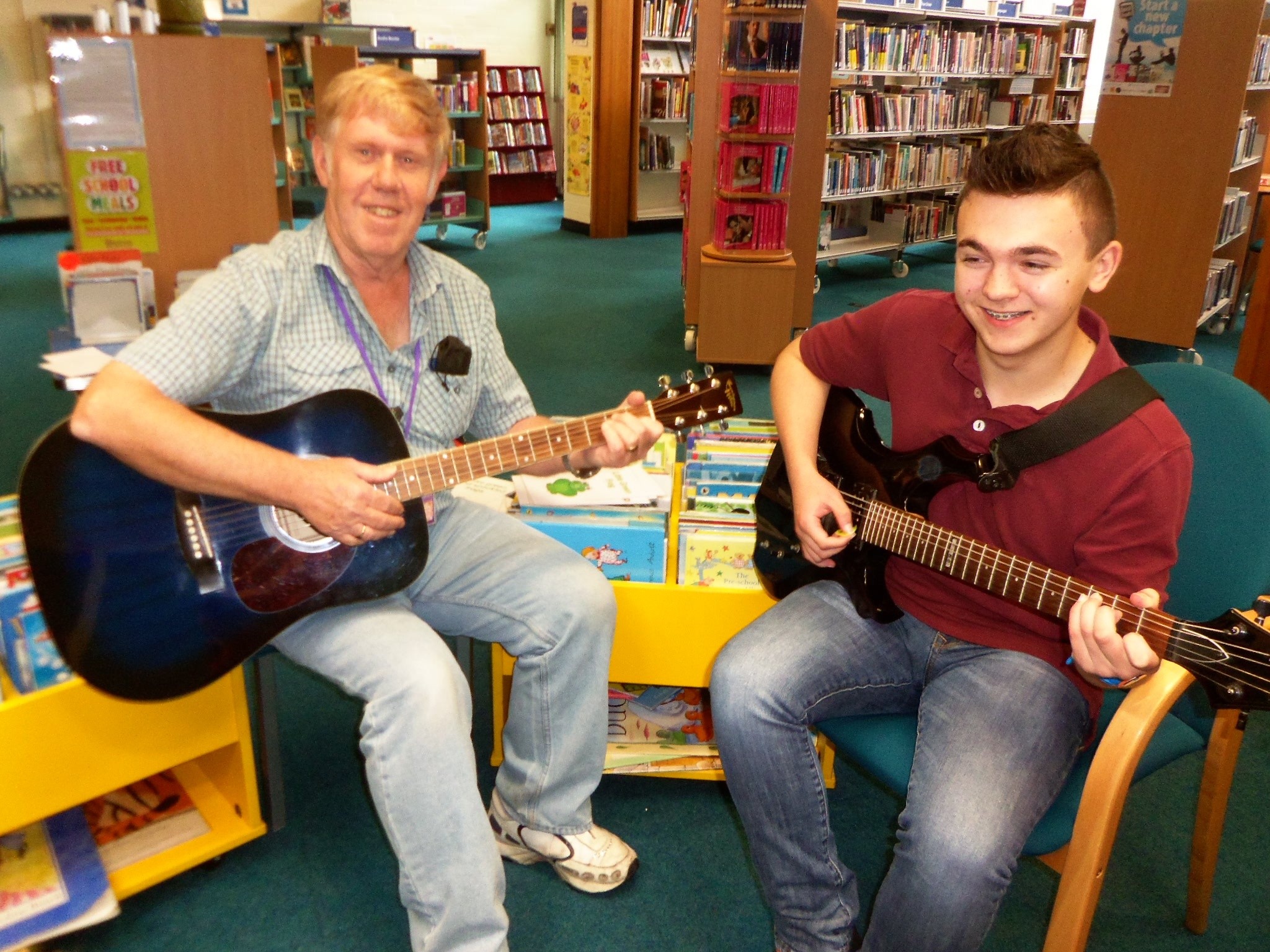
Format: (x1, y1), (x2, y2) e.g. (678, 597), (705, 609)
(46, 0), (1270, 400)
(0, 649), (268, 952)
(489, 578), (836, 786)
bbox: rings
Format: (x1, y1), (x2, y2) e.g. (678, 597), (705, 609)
(627, 445), (638, 452)
(356, 523), (366, 540)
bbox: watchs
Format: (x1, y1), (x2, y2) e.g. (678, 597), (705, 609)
(1065, 651), (1148, 689)
(559, 454), (601, 480)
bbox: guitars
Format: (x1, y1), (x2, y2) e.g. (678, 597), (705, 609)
(11, 363), (746, 707)
(747, 383), (1270, 733)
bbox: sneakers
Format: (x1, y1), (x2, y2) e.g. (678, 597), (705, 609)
(486, 787), (640, 893)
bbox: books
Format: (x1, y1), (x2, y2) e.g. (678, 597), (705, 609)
(714, 0), (806, 253)
(37, 249), (216, 393)
(451, 410), (798, 594)
(270, 35), (332, 189)
(0, 491), (214, 952)
(638, 0), (696, 170)
(818, 18), (1089, 251)
(600, 681), (814, 776)
(485, 66), (557, 174)
(356, 52), (479, 220)
(1196, 34), (1270, 320)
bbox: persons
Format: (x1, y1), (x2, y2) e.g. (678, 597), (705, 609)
(742, 21), (767, 57)
(727, 215), (752, 244)
(819, 212), (831, 250)
(702, 117), (1194, 952)
(67, 60), (652, 952)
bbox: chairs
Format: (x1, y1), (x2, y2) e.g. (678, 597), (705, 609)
(818, 362), (1270, 952)
